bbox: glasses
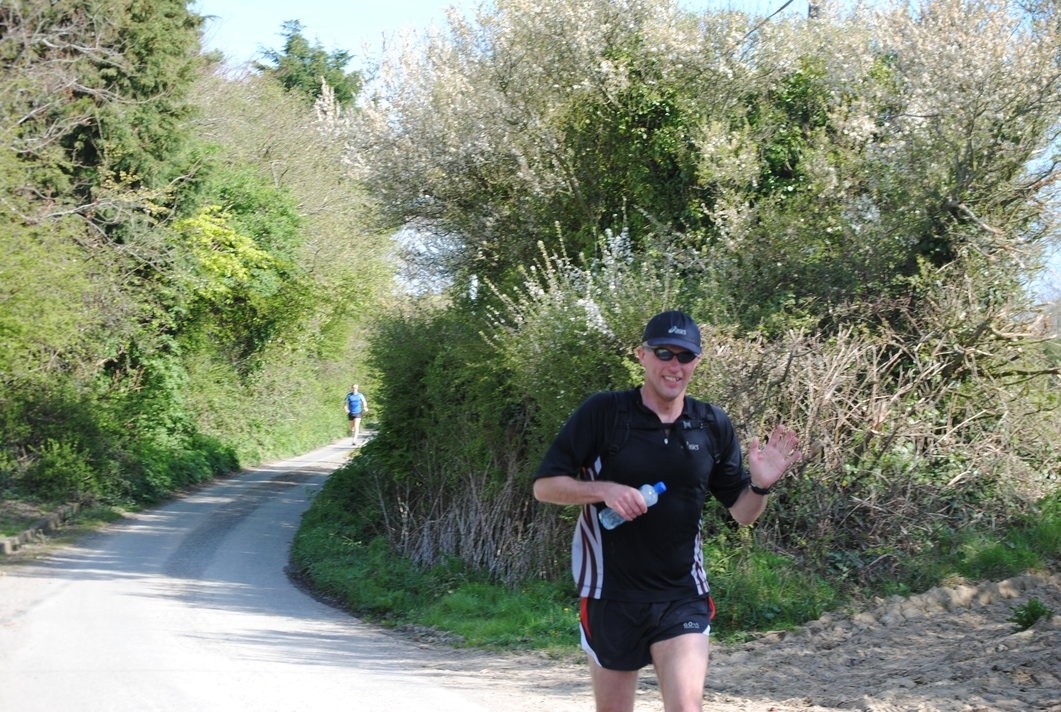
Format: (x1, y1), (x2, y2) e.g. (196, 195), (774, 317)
(646, 345), (694, 364)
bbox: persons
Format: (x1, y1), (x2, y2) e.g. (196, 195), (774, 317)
(343, 383), (369, 445)
(534, 309), (799, 712)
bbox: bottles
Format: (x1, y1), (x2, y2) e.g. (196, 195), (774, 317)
(598, 482), (667, 530)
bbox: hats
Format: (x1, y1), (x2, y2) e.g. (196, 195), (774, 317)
(642, 311), (702, 354)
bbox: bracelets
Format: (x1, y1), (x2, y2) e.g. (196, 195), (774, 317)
(746, 478), (775, 499)
